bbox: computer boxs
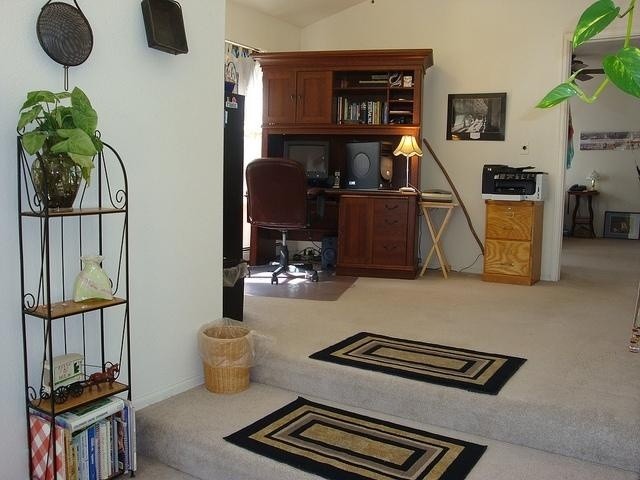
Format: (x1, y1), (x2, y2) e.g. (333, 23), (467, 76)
(320, 234), (338, 274)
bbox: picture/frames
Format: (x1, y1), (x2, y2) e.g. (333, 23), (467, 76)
(446, 92), (507, 141)
(603, 211), (640, 240)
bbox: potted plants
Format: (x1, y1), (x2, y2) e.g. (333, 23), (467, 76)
(18, 87), (103, 213)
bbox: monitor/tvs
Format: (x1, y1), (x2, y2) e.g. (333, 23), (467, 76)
(282, 140), (331, 191)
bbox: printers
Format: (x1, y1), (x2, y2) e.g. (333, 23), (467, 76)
(481, 163), (552, 203)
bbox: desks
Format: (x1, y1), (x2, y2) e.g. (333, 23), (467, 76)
(567, 189), (599, 240)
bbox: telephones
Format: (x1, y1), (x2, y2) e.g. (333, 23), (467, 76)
(332, 168), (343, 188)
(569, 184), (586, 191)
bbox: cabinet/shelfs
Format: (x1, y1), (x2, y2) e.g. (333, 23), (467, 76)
(222, 92), (245, 321)
(17, 131), (136, 480)
(483, 200), (544, 286)
(249, 188), (420, 280)
(250, 48), (434, 192)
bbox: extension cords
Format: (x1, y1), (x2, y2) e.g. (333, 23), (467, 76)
(294, 250), (314, 261)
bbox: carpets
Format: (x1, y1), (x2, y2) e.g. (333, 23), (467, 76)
(222, 395), (488, 480)
(308, 332), (528, 396)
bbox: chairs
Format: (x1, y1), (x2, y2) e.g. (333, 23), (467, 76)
(246, 158), (324, 285)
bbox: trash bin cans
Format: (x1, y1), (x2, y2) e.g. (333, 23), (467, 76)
(223, 256), (250, 323)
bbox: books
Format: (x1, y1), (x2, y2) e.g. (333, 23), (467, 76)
(27, 394), (136, 480)
(390, 111), (410, 114)
(333, 95), (387, 126)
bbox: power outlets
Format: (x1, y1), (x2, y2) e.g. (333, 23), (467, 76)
(520, 141), (529, 155)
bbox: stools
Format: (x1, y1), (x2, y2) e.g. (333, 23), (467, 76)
(418, 202), (459, 280)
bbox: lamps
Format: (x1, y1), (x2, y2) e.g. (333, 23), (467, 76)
(393, 136), (423, 193)
(587, 170), (601, 191)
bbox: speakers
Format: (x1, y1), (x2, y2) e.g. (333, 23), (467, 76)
(140, 0), (190, 55)
(341, 140), (396, 191)
